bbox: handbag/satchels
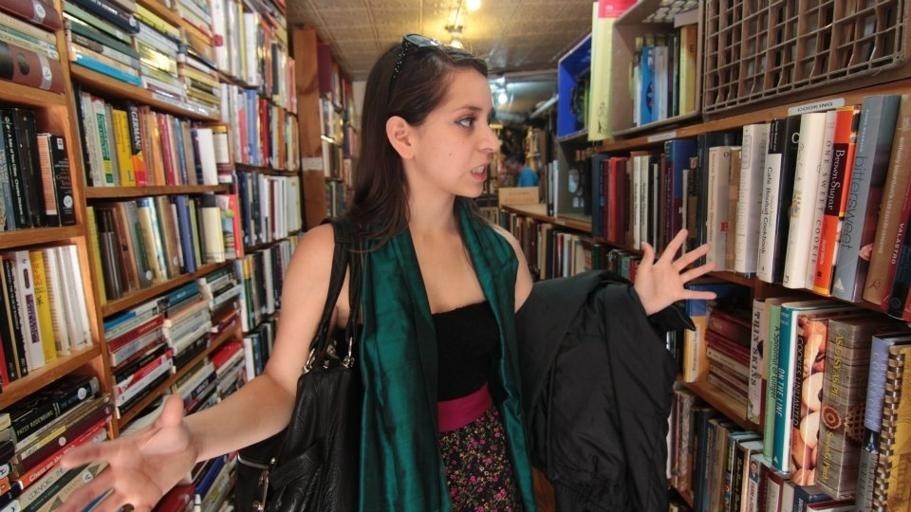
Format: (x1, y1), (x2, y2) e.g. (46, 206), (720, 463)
(235, 337), (353, 511)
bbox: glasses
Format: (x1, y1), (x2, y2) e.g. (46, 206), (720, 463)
(387, 34), (441, 104)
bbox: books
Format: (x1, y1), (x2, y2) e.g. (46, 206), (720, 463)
(547, 26), (911, 325)
(2, 2), (302, 510)
(479, 204), (552, 283)
(483, 125), (506, 171)
(315, 36), (362, 219)
(666, 324), (910, 512)
(522, 126), (544, 168)
(479, 171), (519, 197)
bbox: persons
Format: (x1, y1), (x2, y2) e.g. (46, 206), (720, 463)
(49, 32), (720, 512)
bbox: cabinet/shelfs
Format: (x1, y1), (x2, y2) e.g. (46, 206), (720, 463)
(470, 0), (910, 512)
(290, 27), (361, 230)
(1, 1), (304, 512)
